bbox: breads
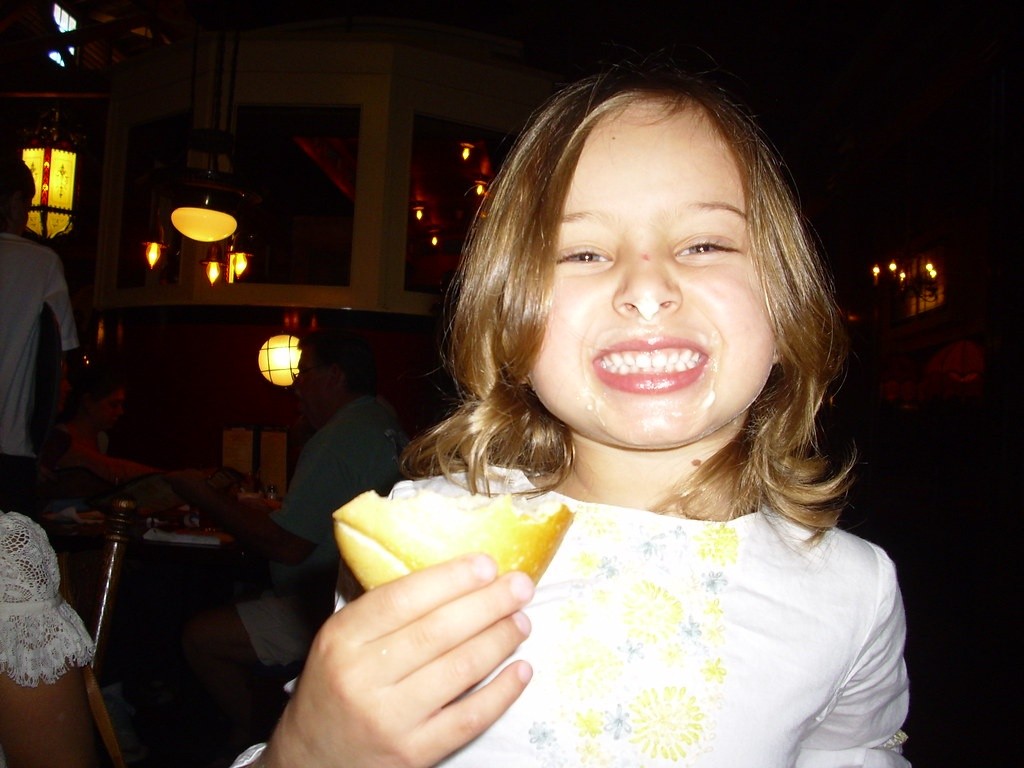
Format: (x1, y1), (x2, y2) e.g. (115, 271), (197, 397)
(333, 480), (577, 593)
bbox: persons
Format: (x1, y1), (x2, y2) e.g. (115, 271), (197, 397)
(0, 151), (414, 768)
(247, 63), (915, 767)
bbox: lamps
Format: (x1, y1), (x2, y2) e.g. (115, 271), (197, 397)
(145, 18), (248, 286)
(16, 98), (87, 247)
